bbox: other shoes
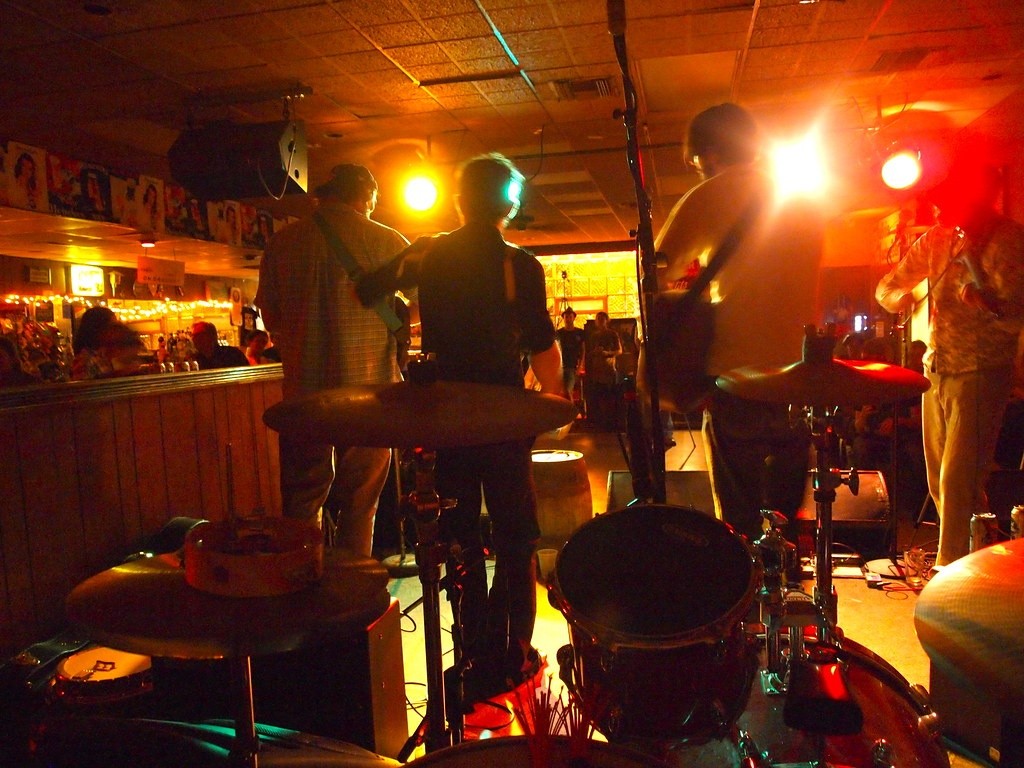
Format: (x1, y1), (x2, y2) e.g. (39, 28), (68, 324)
(665, 438), (676, 452)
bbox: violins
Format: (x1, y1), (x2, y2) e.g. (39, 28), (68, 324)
(947, 207), (999, 307)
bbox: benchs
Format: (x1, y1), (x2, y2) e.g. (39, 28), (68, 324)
(915, 538), (1024, 760)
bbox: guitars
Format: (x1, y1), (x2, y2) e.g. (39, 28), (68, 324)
(391, 290), (411, 372)
(635, 287), (719, 412)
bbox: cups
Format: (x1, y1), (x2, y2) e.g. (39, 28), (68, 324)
(903, 551), (923, 587)
(536, 549), (559, 578)
(158, 361), (200, 372)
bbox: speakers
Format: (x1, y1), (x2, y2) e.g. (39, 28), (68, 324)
(795, 471), (893, 560)
(608, 469), (715, 518)
(166, 118), (308, 201)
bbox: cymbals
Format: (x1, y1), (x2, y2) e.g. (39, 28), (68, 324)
(262, 383), (578, 450)
(714, 356), (931, 408)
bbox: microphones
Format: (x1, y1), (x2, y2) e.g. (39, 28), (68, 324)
(607, 0), (627, 37)
(397, 718), (426, 761)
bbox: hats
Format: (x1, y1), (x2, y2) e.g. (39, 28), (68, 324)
(314, 163), (378, 198)
(562, 307), (576, 319)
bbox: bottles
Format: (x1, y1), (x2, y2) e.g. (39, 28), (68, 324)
(167, 330), (190, 354)
(735, 731), (776, 768)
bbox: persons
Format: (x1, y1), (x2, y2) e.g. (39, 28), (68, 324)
(75, 103), (1024, 701)
(5, 152), (274, 249)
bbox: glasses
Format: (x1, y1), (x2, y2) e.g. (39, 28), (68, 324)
(189, 332), (210, 338)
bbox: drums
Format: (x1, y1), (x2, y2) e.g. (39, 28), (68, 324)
(609, 625), (953, 768)
(550, 505), (759, 743)
(401, 735), (665, 768)
(42, 644), (155, 718)
